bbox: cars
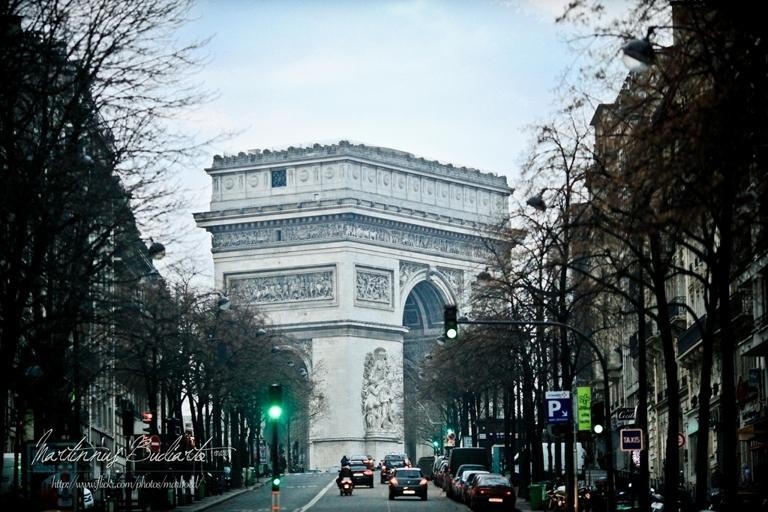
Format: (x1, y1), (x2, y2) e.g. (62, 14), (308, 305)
(338, 446), (516, 512)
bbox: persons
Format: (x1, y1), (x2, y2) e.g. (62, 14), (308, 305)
(336, 456), (354, 488)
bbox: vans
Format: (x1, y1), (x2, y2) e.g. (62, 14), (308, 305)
(542, 442), (600, 469)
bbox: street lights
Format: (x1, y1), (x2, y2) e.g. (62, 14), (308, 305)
(621, 26), (721, 104)
(89, 243), (281, 365)
(477, 265), (515, 280)
(528, 188), (589, 211)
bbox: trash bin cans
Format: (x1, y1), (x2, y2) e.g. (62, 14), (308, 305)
(246, 467), (257, 486)
(529, 484), (548, 511)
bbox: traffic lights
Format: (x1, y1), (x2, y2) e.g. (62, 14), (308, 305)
(446, 424), (455, 434)
(272, 476), (280, 489)
(444, 305), (458, 340)
(433, 432), (442, 448)
(591, 400), (604, 434)
(269, 384), (282, 420)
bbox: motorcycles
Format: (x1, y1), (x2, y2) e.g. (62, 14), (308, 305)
(546, 465), (720, 512)
